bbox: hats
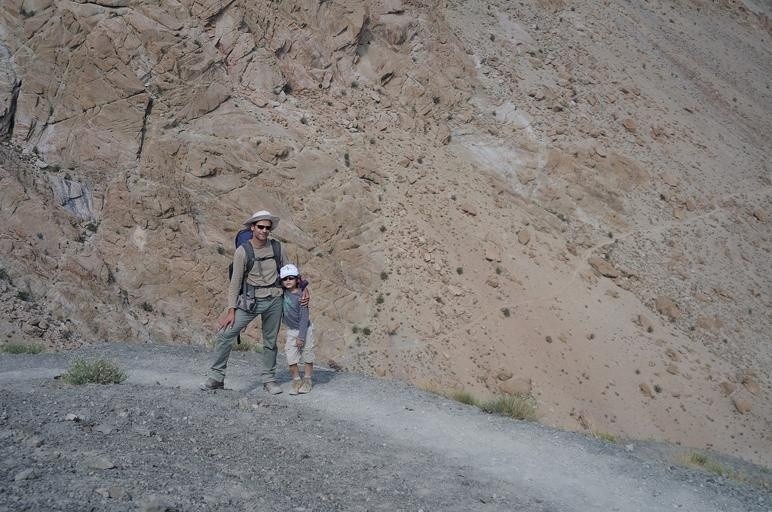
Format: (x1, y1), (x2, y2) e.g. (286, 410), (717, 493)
(243, 211), (280, 231)
(280, 264), (299, 279)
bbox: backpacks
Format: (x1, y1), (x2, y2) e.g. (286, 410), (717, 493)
(229, 229), (280, 295)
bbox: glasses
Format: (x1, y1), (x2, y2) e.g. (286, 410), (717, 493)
(256, 225), (272, 230)
(282, 276), (295, 281)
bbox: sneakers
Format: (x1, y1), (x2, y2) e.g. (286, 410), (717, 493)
(289, 379), (312, 395)
(199, 377), (224, 391)
(263, 381), (283, 395)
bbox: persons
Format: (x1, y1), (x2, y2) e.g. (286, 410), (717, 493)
(199, 210), (310, 395)
(279, 262), (315, 394)
